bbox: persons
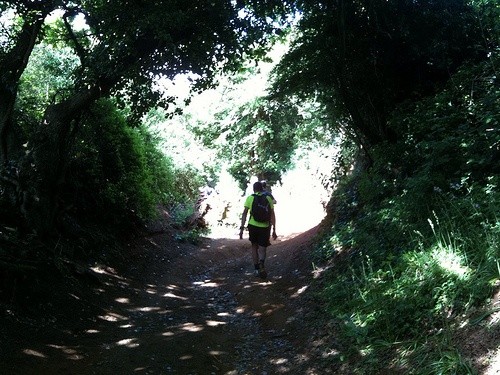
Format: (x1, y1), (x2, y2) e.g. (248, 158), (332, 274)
(238, 182), (277, 277)
(260, 180), (278, 248)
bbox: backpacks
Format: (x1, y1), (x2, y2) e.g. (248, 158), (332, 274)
(250, 193), (271, 223)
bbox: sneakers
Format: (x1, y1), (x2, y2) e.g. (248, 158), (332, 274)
(253, 272), (259, 277)
(258, 262), (267, 278)
